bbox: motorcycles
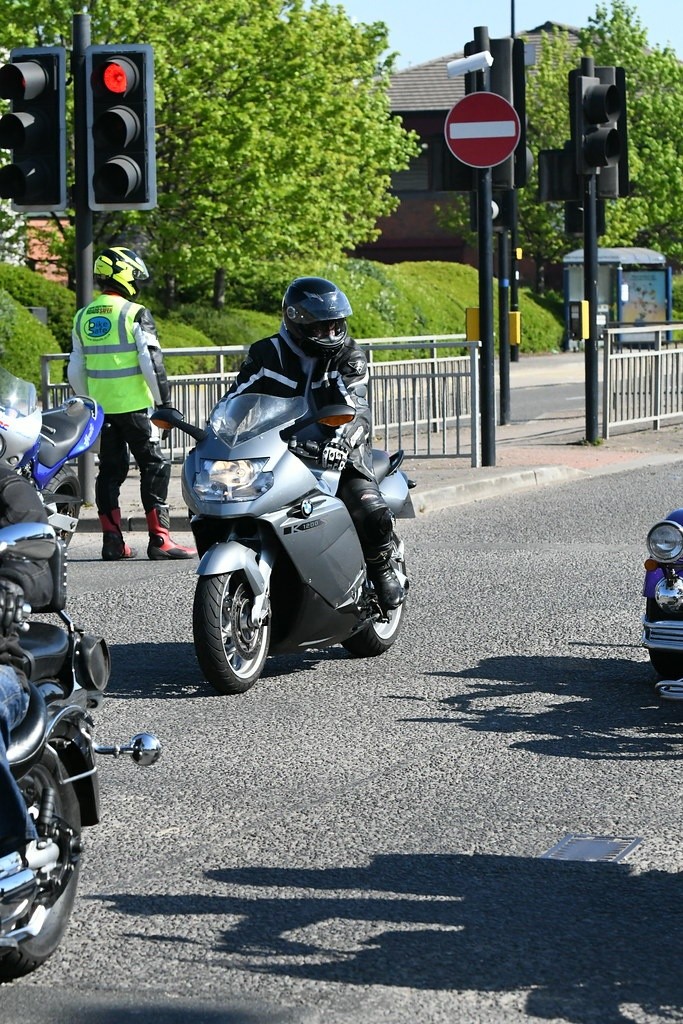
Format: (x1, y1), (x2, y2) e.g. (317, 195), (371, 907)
(1, 365), (107, 551)
(0, 523), (168, 982)
(150, 390), (422, 693)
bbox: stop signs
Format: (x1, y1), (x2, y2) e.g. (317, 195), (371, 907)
(445, 90), (523, 171)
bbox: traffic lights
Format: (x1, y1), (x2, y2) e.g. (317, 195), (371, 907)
(84, 40), (159, 213)
(470, 188), (515, 230)
(574, 76), (628, 175)
(564, 199), (607, 238)
(0, 46), (70, 214)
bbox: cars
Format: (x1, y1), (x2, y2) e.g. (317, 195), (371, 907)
(640, 505), (683, 703)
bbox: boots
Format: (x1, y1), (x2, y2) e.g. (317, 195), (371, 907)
(98, 506), (140, 562)
(145, 507), (198, 560)
(363, 537), (405, 610)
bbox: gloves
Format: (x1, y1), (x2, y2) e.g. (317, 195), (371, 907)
(320, 437), (350, 471)
(156, 402), (173, 440)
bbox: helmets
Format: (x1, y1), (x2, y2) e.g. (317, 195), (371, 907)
(93, 247), (149, 303)
(281, 277), (352, 360)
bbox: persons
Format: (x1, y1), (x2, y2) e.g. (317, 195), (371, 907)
(69, 245), (201, 568)
(0, 459), (65, 927)
(221, 274), (416, 608)
